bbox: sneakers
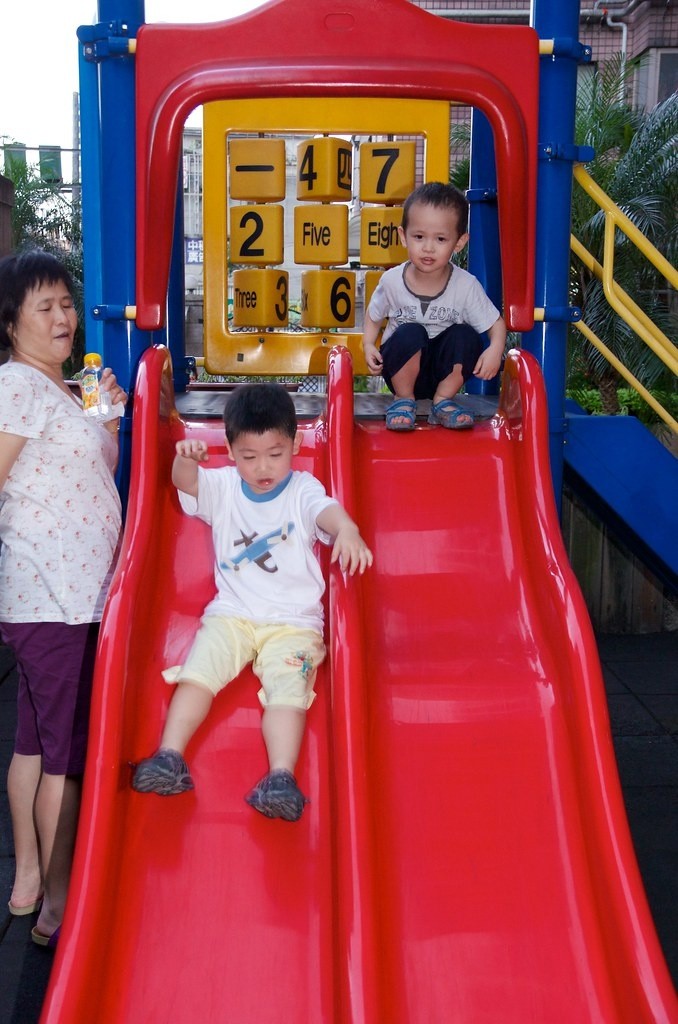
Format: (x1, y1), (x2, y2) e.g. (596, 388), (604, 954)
(243, 768), (311, 823)
(125, 748), (196, 796)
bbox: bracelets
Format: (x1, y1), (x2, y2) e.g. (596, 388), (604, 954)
(110, 424), (120, 434)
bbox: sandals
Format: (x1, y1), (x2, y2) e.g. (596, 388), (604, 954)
(427, 399), (477, 429)
(385, 397), (418, 432)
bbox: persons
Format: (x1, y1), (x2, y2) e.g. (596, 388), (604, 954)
(131, 384), (375, 822)
(0, 250), (127, 948)
(363, 182), (506, 431)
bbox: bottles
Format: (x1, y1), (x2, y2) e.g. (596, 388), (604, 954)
(78, 354), (113, 425)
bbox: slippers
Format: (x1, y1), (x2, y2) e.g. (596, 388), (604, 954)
(7, 895), (44, 916)
(31, 924), (51, 946)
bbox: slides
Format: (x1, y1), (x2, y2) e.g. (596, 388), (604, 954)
(41, 343), (678, 1024)
